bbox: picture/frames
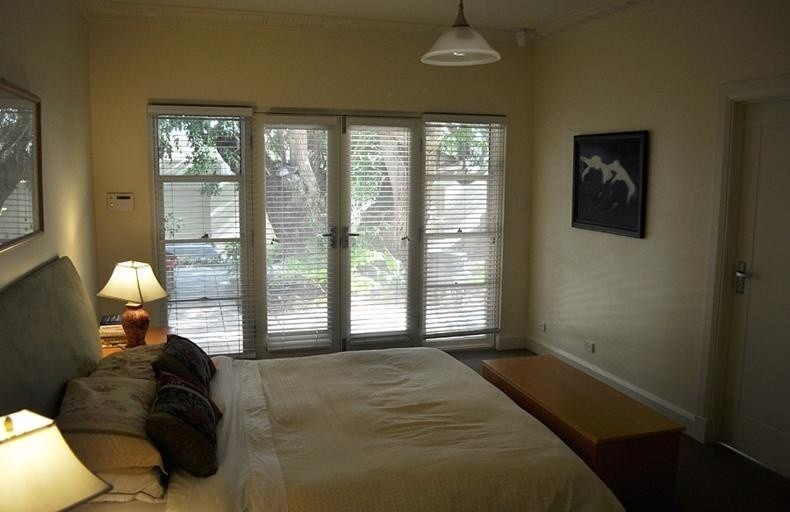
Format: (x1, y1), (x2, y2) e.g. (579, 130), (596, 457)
(0, 74), (46, 259)
(569, 128), (651, 241)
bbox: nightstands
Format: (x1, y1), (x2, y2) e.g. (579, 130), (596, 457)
(100, 325), (169, 359)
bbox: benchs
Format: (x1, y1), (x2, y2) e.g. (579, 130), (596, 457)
(477, 353), (689, 511)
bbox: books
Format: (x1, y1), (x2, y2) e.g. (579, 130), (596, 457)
(100, 325), (128, 348)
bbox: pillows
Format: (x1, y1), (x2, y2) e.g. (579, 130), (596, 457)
(88, 343), (163, 379)
(89, 471), (170, 506)
(149, 333), (220, 392)
(51, 374), (171, 476)
(143, 367), (224, 478)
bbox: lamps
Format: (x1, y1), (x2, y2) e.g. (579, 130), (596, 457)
(0, 406), (116, 512)
(418, 1), (503, 68)
(95, 258), (174, 348)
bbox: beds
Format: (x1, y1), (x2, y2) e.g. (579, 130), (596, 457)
(0, 254), (631, 511)
